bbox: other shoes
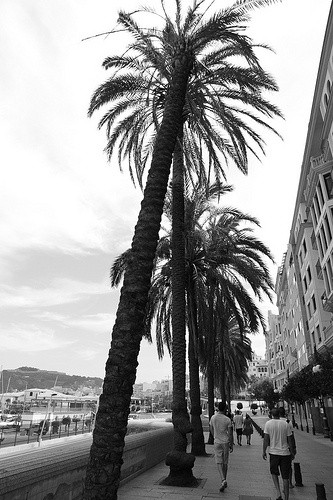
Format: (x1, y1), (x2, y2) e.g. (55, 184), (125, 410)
(237, 443), (242, 446)
(247, 443), (251, 445)
(219, 480), (226, 492)
(276, 498), (283, 500)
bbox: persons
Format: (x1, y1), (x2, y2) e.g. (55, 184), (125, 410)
(233, 410), (243, 446)
(209, 402), (233, 492)
(275, 407), (296, 489)
(262, 407), (294, 499)
(242, 414), (253, 446)
